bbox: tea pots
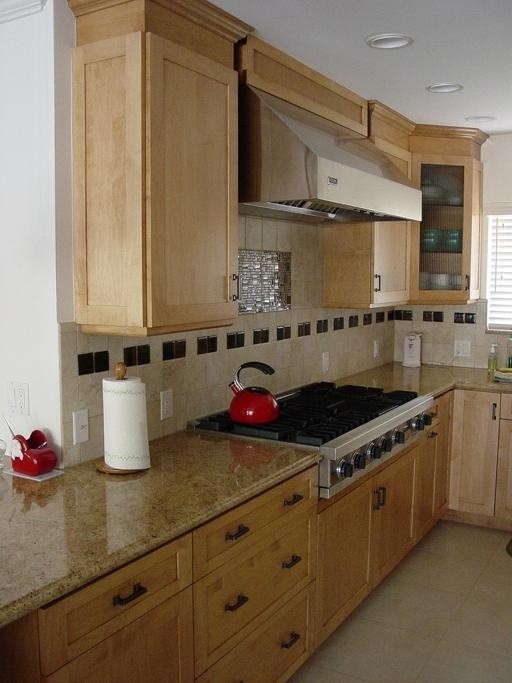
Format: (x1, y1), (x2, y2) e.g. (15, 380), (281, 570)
(227, 361), (281, 425)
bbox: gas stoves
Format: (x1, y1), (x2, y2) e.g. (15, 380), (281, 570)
(185, 381), (435, 498)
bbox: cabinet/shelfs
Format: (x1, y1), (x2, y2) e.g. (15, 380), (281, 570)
(448, 388), (512, 521)
(410, 123), (490, 304)
(414, 426), (449, 531)
(190, 464), (310, 681)
(318, 443), (413, 628)
(323, 221), (412, 308)
(64, 2), (256, 337)
(38, 533), (190, 681)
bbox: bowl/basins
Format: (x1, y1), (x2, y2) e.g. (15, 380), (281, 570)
(420, 183), (463, 290)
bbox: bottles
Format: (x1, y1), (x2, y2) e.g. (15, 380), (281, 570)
(506, 336), (512, 367)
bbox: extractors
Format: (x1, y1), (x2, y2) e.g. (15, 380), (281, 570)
(238, 83), (422, 229)
